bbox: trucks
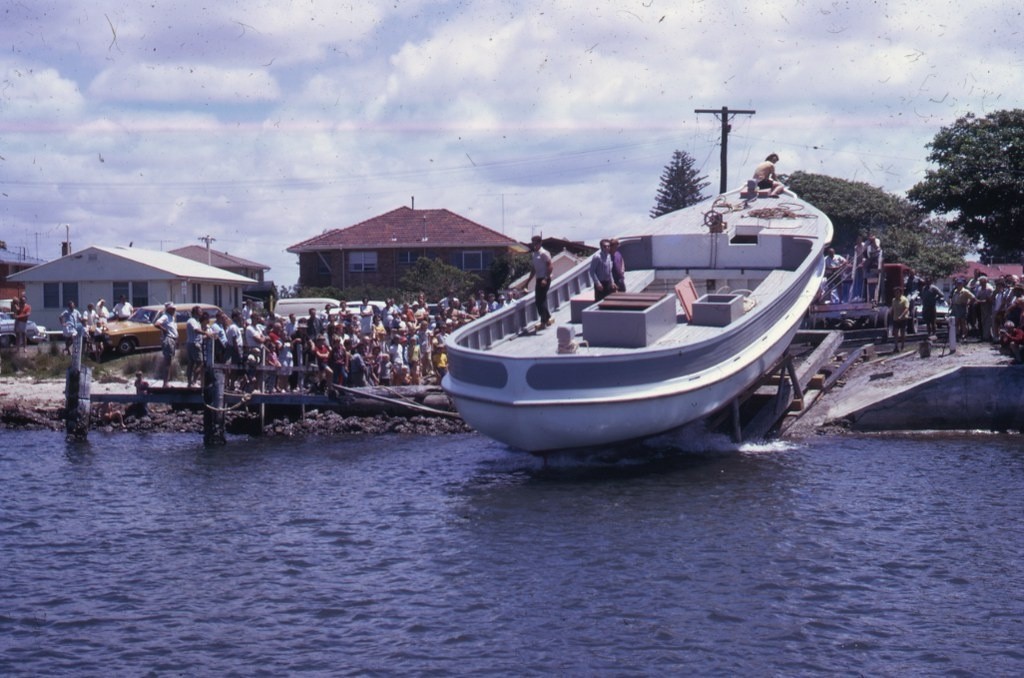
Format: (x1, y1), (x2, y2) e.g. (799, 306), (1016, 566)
(809, 261), (918, 334)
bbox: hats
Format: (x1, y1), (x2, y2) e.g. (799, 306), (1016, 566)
(413, 300), (418, 306)
(165, 302), (176, 311)
(956, 279), (963, 284)
(400, 336), (408, 343)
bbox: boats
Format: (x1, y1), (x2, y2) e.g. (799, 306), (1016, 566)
(442, 181), (834, 460)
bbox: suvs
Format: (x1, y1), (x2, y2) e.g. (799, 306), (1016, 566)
(274, 298), (384, 325)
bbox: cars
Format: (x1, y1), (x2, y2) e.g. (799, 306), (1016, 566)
(0, 313), (46, 348)
(101, 302), (235, 354)
(915, 299), (950, 328)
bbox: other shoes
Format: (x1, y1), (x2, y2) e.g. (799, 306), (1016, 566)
(1010, 360), (1023, 365)
(534, 323), (545, 331)
(545, 318), (555, 326)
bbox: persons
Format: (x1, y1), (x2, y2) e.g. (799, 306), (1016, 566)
(10, 291), (529, 392)
(590, 239), (617, 302)
(609, 239), (626, 292)
(825, 232), (1024, 364)
(754, 153), (784, 198)
(522, 235), (555, 330)
